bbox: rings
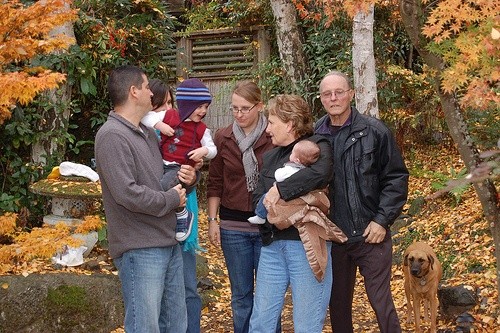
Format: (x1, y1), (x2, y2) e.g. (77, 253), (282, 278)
(265, 205), (271, 208)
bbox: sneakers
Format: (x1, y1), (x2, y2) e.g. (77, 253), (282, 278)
(175, 211), (194, 241)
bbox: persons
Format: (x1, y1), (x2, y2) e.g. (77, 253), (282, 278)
(141, 78), (218, 243)
(95, 65), (202, 333)
(313, 71), (410, 333)
(206, 82), (281, 333)
(145, 78), (202, 328)
(248, 94), (333, 333)
(248, 140), (322, 224)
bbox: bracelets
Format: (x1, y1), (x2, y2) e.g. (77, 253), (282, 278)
(208, 218), (217, 221)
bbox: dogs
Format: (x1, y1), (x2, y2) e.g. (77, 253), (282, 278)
(402, 242), (442, 333)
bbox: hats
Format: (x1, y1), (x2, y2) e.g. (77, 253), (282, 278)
(176, 78), (212, 121)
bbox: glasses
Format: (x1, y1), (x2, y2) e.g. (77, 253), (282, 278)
(229, 103), (258, 114)
(320, 89), (350, 98)
